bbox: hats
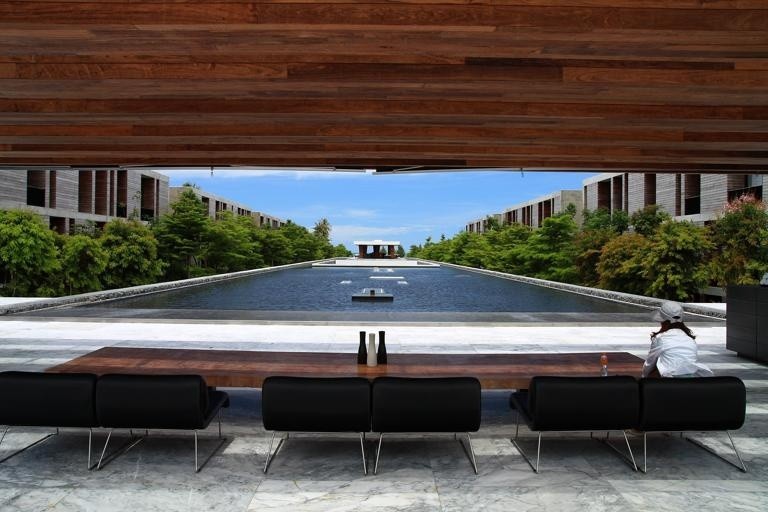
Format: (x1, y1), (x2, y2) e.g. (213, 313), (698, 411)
(654, 301), (683, 323)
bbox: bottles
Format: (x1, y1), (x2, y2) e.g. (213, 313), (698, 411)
(600, 351), (609, 376)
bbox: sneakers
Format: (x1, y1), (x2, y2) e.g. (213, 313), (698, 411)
(627, 429), (644, 438)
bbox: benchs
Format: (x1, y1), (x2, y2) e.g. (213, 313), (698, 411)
(0, 370), (231, 474)
(261, 375), (481, 476)
(510, 374), (747, 473)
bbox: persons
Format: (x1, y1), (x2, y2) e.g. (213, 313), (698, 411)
(623, 300), (698, 437)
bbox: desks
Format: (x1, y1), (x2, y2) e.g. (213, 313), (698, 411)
(46, 346), (644, 389)
